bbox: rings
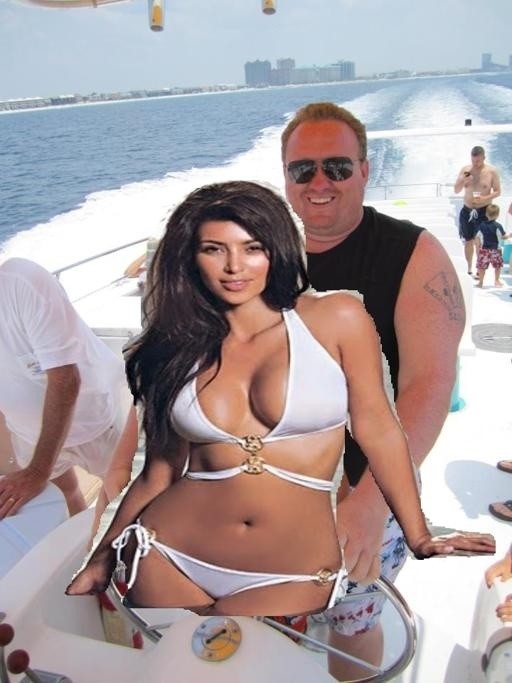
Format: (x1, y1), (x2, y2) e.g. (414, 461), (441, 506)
(7, 497), (19, 506)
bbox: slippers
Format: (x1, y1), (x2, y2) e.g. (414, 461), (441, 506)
(497, 458), (512, 472)
(488, 499), (512, 521)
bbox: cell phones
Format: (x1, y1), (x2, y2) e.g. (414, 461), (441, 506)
(463, 171), (469, 177)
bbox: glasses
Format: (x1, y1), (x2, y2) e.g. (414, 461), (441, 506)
(285, 155), (354, 184)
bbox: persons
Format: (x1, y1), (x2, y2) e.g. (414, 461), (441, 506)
(467, 202), (511, 289)
(486, 459), (512, 523)
(56, 178), (498, 624)
(266, 101), (471, 682)
(1, 257), (140, 526)
(481, 541), (512, 624)
(452, 144), (502, 278)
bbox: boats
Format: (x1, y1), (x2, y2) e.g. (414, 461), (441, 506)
(1, 178), (512, 682)
